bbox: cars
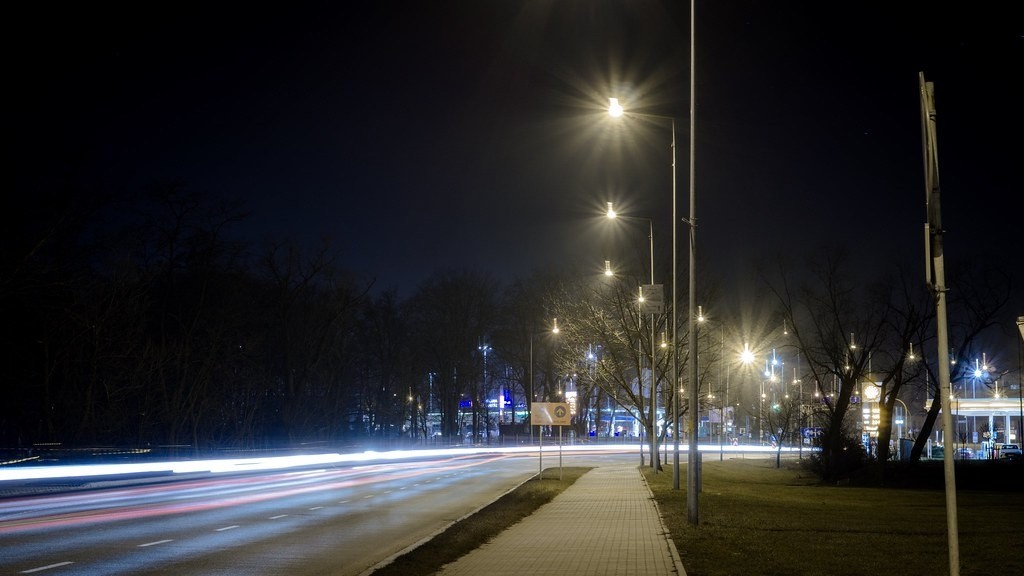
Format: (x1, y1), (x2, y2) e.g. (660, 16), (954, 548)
(931, 442), (1022, 459)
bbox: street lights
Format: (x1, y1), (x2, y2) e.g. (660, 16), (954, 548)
(529, 317), (561, 448)
(606, 202), (661, 473)
(608, 96), (680, 491)
(726, 352), (753, 443)
(606, 261), (645, 470)
